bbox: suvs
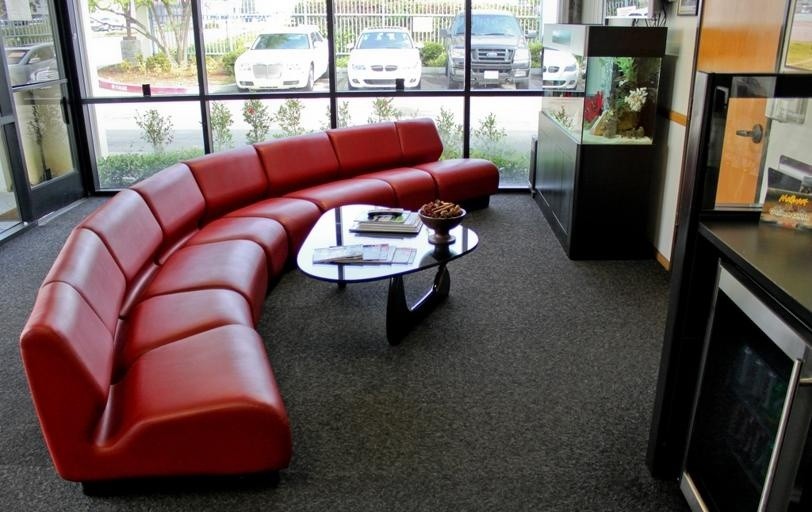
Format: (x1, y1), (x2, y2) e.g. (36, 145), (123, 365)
(439, 9), (540, 89)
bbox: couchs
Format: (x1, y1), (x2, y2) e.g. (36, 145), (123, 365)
(15, 95), (503, 498)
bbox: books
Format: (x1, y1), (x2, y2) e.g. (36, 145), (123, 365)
(349, 206), (424, 235)
(312, 242), (417, 265)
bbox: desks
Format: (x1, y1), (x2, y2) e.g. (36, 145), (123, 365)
(293, 202), (480, 348)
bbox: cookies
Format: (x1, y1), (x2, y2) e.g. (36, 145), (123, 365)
(422, 200), (461, 217)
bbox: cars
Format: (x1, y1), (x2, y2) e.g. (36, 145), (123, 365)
(344, 24), (425, 89)
(543, 43), (579, 91)
(88, 14), (124, 32)
(3, 40), (59, 101)
(235, 24), (328, 92)
(627, 8), (650, 17)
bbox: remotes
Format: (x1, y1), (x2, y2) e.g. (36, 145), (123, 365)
(368, 208), (404, 217)
